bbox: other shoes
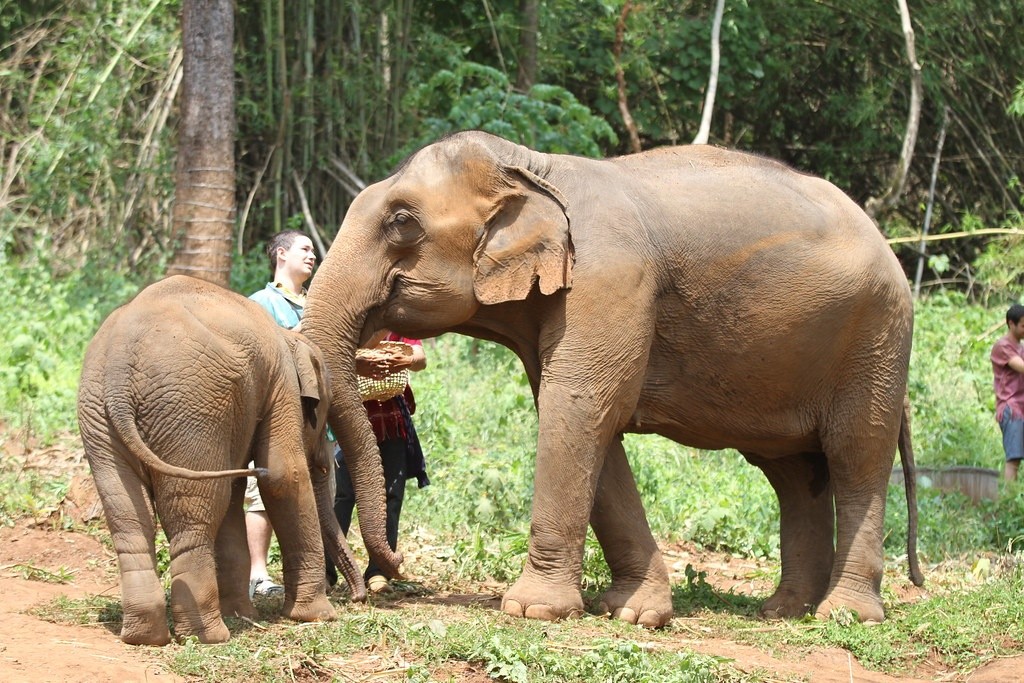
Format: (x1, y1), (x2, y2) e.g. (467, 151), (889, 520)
(248, 577), (286, 597)
(365, 575), (390, 593)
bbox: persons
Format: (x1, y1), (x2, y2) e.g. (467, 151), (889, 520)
(243, 230), (316, 598)
(991, 305), (1024, 485)
(325, 333), (425, 594)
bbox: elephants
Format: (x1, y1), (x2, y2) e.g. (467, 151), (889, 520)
(75, 129), (922, 644)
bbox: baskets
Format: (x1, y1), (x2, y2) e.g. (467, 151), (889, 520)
(355, 341), (414, 402)
